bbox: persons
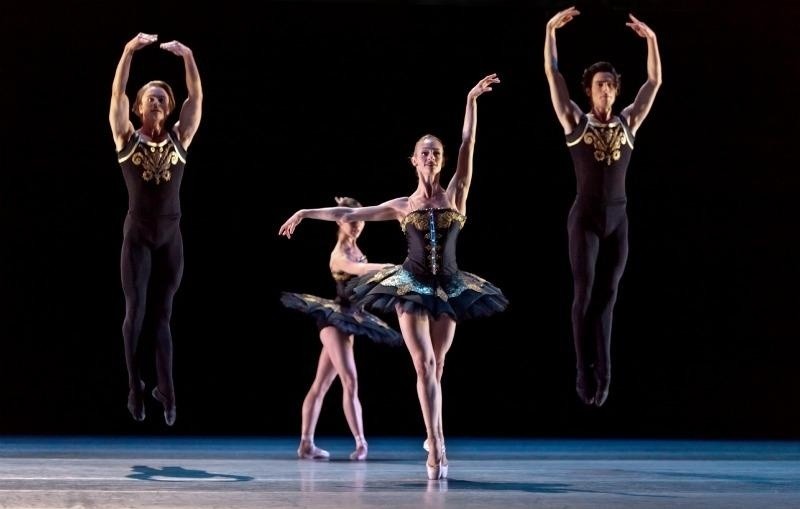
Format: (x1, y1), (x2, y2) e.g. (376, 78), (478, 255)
(545, 5), (662, 407)
(282, 196), (404, 462)
(277, 73), (510, 478)
(107, 33), (204, 426)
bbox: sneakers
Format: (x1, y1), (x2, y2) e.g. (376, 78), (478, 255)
(127, 378), (146, 420)
(297, 446), (330, 459)
(152, 385), (177, 426)
(575, 362), (611, 407)
(423, 439), (448, 480)
(350, 441), (368, 460)
